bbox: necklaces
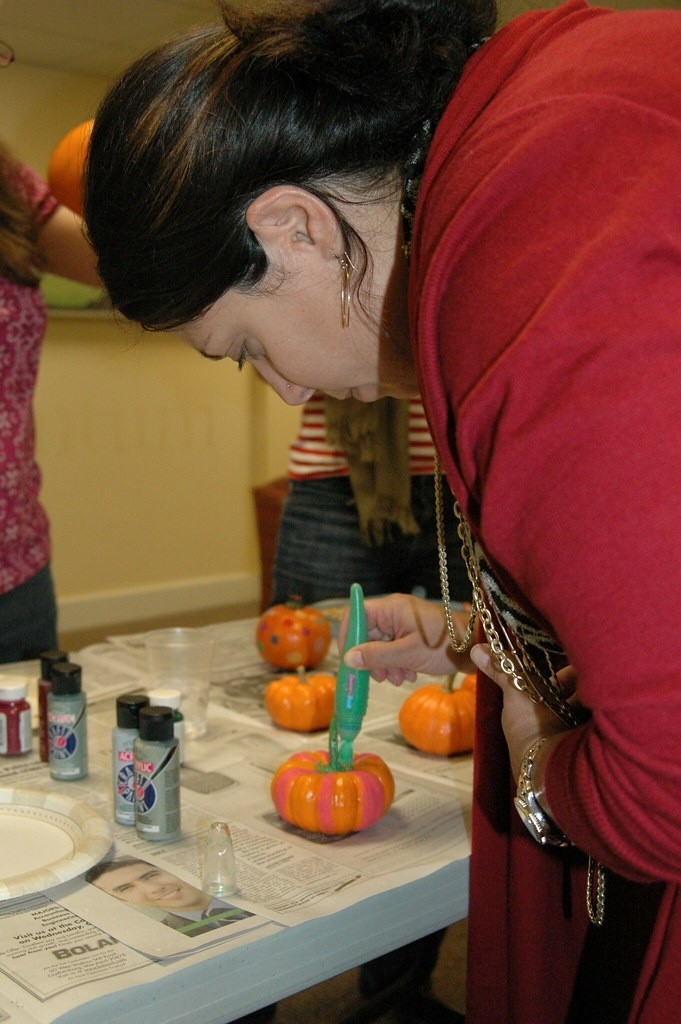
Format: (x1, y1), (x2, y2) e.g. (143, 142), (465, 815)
(428, 448), (606, 927)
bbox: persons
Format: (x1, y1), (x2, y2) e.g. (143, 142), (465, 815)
(270, 392), (480, 1024)
(87, 857), (255, 939)
(81, 0), (681, 1024)
(0, 38), (105, 658)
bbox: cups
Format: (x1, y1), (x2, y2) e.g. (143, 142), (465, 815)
(143, 626), (219, 742)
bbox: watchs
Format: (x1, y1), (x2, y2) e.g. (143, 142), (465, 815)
(513, 736), (576, 848)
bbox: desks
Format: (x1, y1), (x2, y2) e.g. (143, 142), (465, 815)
(0, 597), (475, 1024)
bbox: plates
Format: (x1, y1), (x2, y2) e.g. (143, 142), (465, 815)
(0, 782), (113, 899)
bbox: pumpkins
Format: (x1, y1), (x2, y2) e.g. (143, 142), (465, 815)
(401, 672), (476, 756)
(272, 716), (394, 835)
(257, 602), (330, 671)
(266, 666), (336, 731)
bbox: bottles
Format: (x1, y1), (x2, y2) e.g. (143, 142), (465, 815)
(38, 650), (89, 780)
(1, 679), (33, 756)
(113, 689), (185, 842)
